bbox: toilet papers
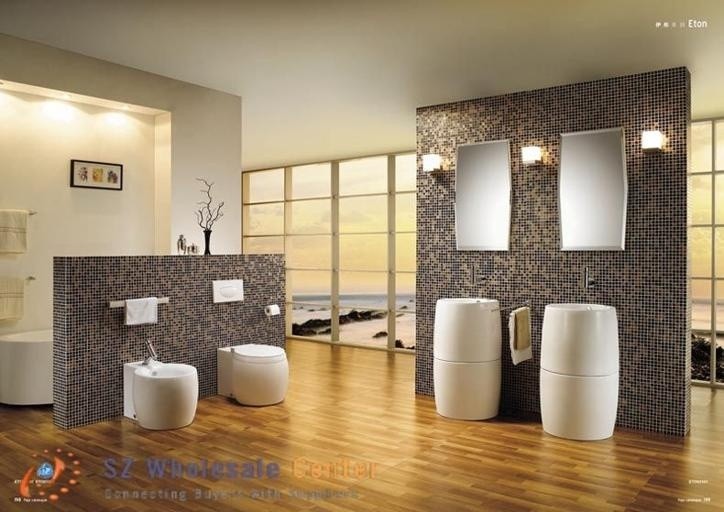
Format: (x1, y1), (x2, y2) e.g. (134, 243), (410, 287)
(265, 304), (281, 316)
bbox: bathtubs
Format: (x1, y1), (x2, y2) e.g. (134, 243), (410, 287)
(0, 329), (53, 406)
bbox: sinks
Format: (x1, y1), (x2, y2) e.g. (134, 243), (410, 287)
(539, 303), (620, 440)
(432, 298), (501, 421)
(122, 360), (199, 430)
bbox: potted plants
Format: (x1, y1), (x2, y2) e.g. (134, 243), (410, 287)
(195, 179), (224, 256)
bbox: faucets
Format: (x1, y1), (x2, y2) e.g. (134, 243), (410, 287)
(143, 339), (157, 366)
(469, 265), (486, 286)
(582, 266), (595, 289)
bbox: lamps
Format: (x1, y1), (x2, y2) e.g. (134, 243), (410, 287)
(520, 146), (543, 168)
(640, 130), (664, 155)
(421, 154), (442, 173)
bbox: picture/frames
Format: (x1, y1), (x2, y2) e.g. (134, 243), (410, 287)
(69, 159), (123, 190)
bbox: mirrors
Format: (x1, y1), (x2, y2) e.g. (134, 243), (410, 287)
(556, 127), (628, 253)
(454, 138), (511, 253)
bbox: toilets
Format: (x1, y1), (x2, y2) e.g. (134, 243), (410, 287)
(216, 343), (288, 407)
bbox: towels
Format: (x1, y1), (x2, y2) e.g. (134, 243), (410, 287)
(0, 209), (30, 256)
(507, 305), (534, 366)
(0, 275), (25, 324)
(124, 297), (159, 327)
(211, 278), (244, 305)
